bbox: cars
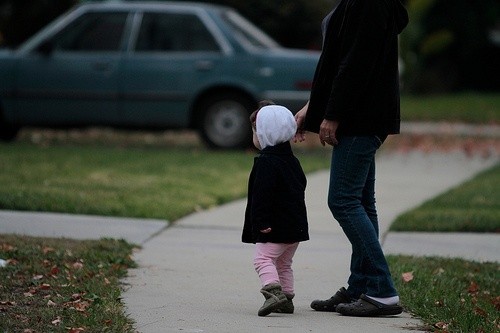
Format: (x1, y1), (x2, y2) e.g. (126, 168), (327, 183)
(0, 0), (322, 144)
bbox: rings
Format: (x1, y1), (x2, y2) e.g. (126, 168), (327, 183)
(325, 135), (330, 138)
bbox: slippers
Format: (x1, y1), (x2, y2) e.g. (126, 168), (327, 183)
(337, 294), (403, 316)
(310, 286), (360, 311)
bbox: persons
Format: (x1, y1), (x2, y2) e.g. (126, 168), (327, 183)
(241, 100), (310, 317)
(293, 0), (409, 316)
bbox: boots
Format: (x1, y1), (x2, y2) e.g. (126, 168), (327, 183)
(258, 284), (287, 316)
(271, 292), (295, 313)
